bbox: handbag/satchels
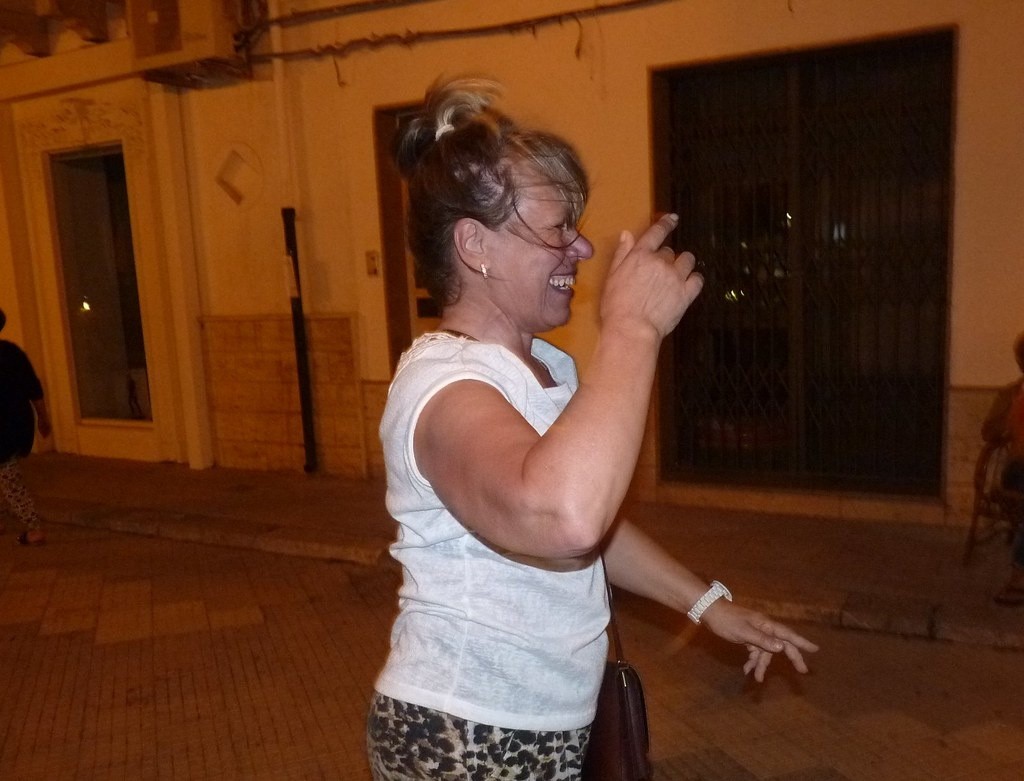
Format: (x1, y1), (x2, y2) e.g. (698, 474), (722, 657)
(583, 661), (655, 781)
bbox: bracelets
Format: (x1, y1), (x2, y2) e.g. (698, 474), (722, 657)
(688, 580), (732, 624)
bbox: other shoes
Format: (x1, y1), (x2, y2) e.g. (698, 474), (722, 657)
(991, 583), (1024, 606)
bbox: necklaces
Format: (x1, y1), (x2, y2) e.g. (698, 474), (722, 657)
(444, 329), (476, 341)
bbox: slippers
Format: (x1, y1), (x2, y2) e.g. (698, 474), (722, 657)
(15, 532), (44, 547)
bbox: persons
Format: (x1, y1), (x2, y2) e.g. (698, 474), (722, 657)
(0, 311), (53, 546)
(977, 334), (1024, 607)
(366, 82), (819, 781)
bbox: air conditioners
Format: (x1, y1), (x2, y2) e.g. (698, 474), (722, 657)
(125, 0), (254, 83)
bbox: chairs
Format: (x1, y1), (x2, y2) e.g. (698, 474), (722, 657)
(962, 437), (1023, 562)
